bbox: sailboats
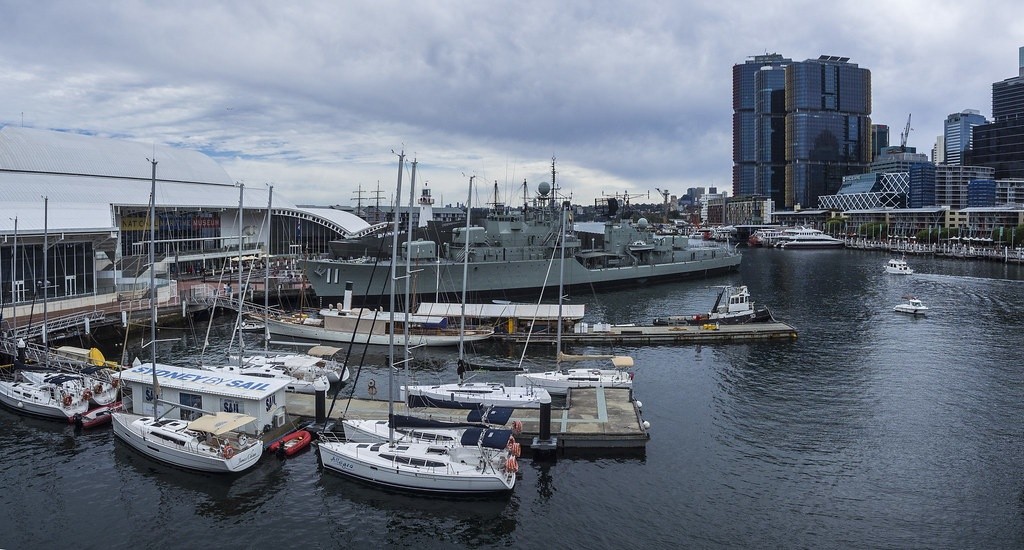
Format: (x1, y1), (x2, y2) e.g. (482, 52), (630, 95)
(316, 155), (517, 496)
(514, 205), (633, 397)
(342, 159), (521, 458)
(399, 171), (551, 409)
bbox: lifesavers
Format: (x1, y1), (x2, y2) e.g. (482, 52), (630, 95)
(84, 389), (92, 400)
(507, 436), (522, 457)
(507, 458), (519, 473)
(112, 380), (117, 388)
(64, 395), (72, 406)
(224, 445), (234, 459)
(95, 385), (101, 394)
(512, 420), (523, 432)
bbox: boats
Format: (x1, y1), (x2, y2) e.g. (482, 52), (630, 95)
(194, 184), (331, 396)
(886, 257), (914, 275)
(236, 319), (265, 334)
(72, 400), (126, 431)
(0, 216), (117, 405)
(228, 187), (350, 383)
(251, 282), (495, 346)
(747, 224), (845, 249)
(650, 218), (737, 241)
(296, 151), (742, 311)
(653, 284), (772, 326)
(893, 296), (928, 315)
(1, 193), (89, 423)
(110, 160), (264, 476)
(272, 431), (313, 459)
